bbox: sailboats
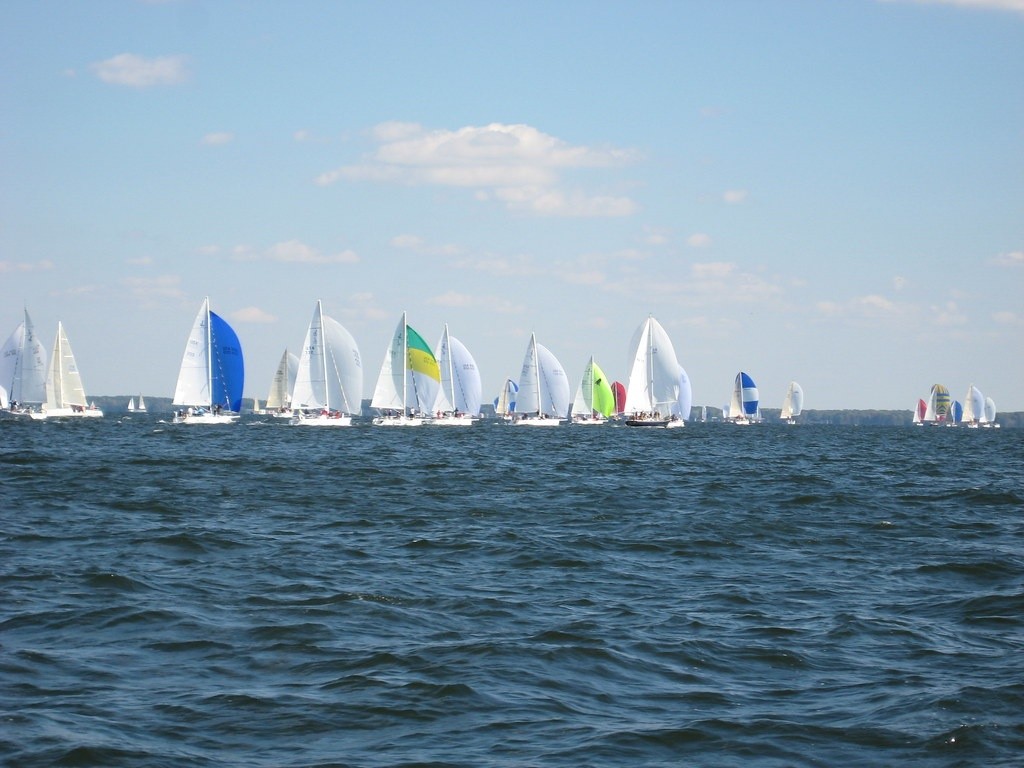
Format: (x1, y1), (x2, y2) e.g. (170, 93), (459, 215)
(780, 382), (804, 425)
(911, 383), (1002, 428)
(0, 295), (771, 427)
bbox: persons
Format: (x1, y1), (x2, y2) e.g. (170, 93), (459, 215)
(9, 399), (37, 416)
(179, 404), (218, 422)
(503, 409), (795, 425)
(281, 404), (459, 419)
(74, 406), (77, 413)
